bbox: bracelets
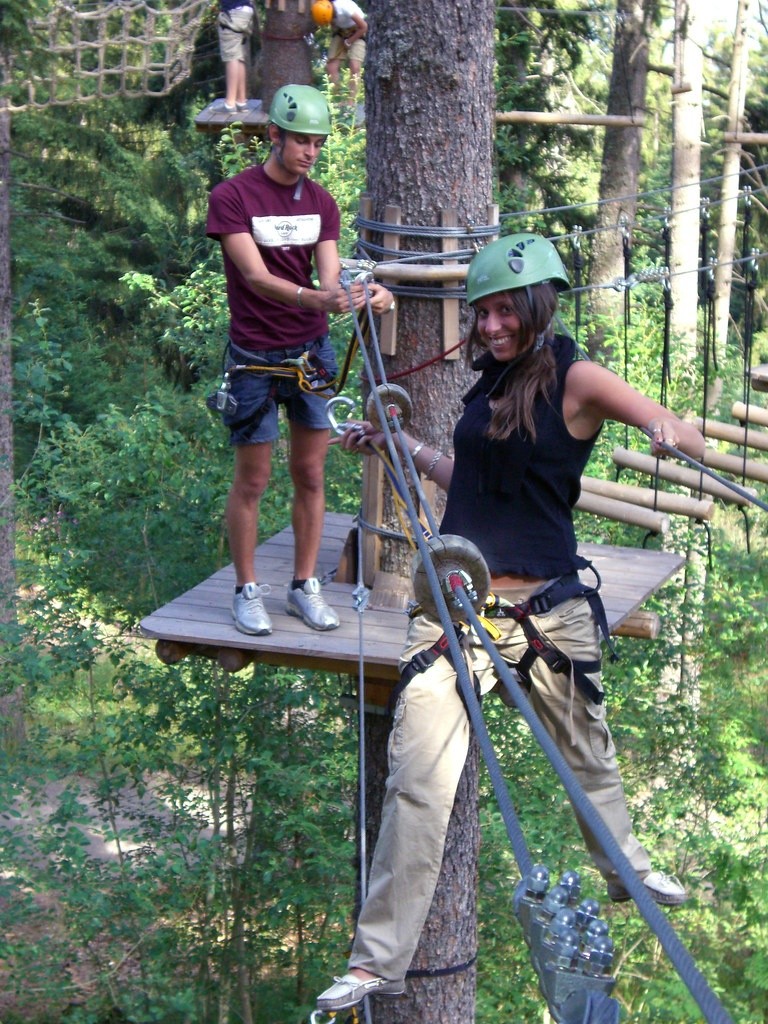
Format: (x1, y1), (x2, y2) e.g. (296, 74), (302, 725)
(406, 442), (424, 458)
(297, 287), (303, 305)
(344, 39), (351, 47)
(390, 301), (395, 310)
(425, 450), (443, 479)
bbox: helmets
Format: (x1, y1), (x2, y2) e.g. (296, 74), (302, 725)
(311, 0), (332, 24)
(269, 85), (332, 134)
(467, 233), (571, 304)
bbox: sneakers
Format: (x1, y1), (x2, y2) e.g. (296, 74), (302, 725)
(231, 583), (272, 634)
(285, 578), (340, 630)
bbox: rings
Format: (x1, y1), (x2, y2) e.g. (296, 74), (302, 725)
(653, 429), (662, 432)
(667, 439), (675, 446)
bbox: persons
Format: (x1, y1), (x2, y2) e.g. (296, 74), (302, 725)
(310, 0), (368, 103)
(316, 234), (706, 1011)
(209, 0), (254, 114)
(204, 84), (396, 636)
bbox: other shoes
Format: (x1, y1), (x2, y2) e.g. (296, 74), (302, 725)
(317, 974), (404, 1010)
(608, 871), (686, 903)
(208, 101), (249, 114)
(343, 101), (355, 118)
